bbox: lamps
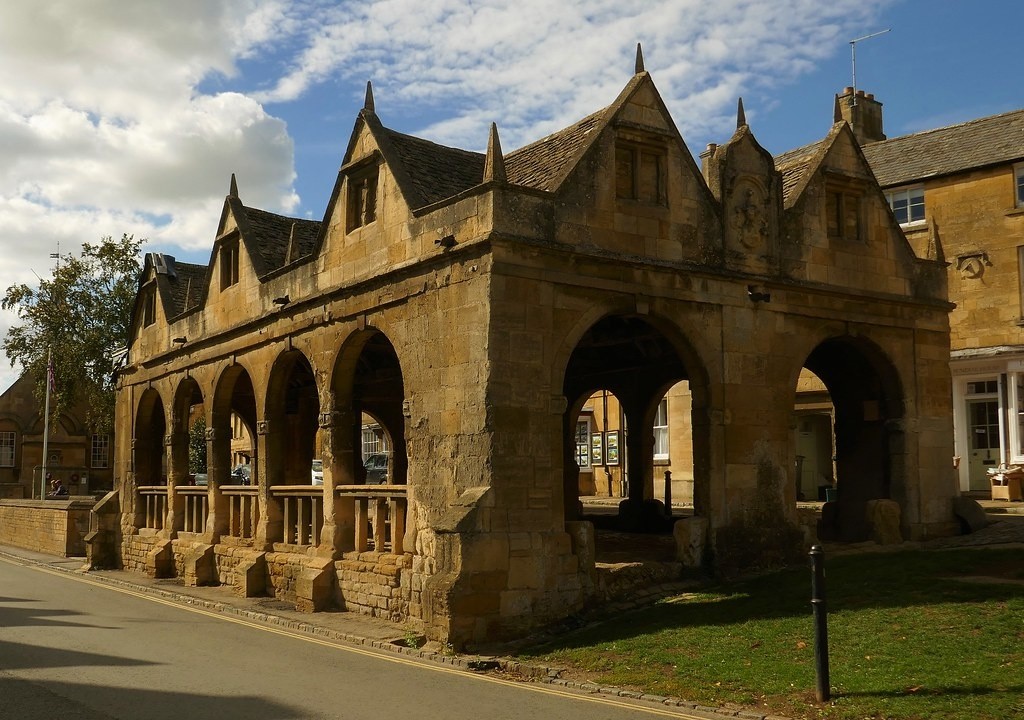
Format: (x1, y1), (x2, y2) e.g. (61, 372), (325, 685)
(953, 456), (962, 469)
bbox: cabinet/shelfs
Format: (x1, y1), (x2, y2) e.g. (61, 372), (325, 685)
(986, 473), (1023, 502)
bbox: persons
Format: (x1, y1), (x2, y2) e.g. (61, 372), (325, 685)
(50, 480), (65, 496)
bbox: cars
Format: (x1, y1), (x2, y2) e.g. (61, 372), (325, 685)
(231, 464), (251, 485)
(311, 459), (323, 485)
(362, 450), (387, 485)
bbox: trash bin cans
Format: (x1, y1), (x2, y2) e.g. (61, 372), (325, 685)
(794, 455), (806, 501)
